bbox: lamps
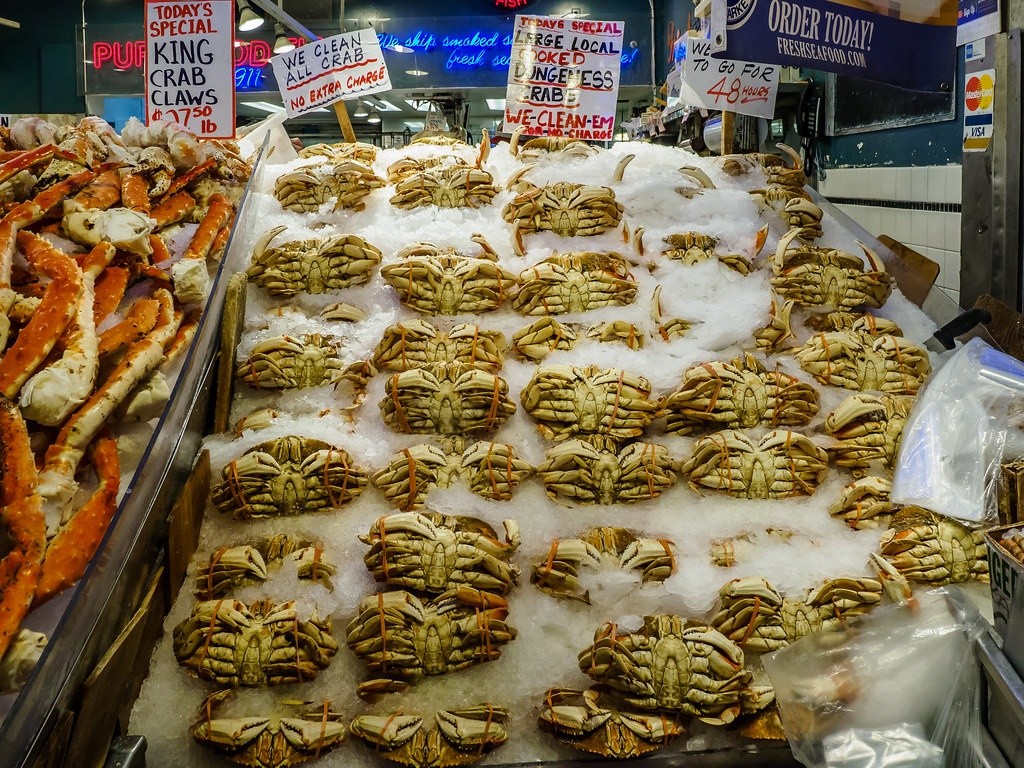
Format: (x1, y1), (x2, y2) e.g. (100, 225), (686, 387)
(236, 0), (266, 32)
(386, 42), (414, 54)
(273, 22), (296, 54)
(353, 100), (368, 117)
(366, 105), (382, 123)
(405, 54), (429, 76)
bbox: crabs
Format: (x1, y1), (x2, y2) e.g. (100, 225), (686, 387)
(0, 112), (247, 671)
(180, 139), (998, 768)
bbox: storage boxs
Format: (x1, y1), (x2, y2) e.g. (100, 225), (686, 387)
(985, 520), (1024, 682)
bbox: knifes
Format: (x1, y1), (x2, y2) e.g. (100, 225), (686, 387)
(923, 309), (991, 354)
